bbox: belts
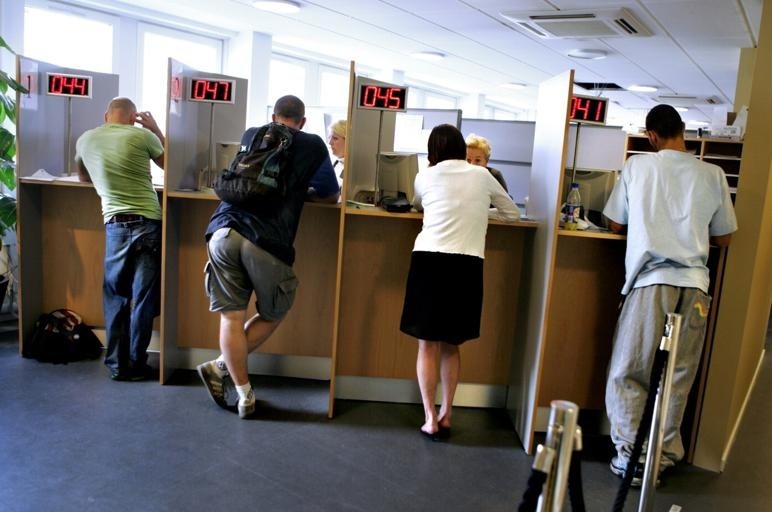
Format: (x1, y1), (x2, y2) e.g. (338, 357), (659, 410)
(109, 213), (145, 224)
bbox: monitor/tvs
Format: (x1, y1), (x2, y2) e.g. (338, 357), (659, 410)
(215, 142), (241, 177)
(561, 167), (617, 228)
(375, 151), (418, 206)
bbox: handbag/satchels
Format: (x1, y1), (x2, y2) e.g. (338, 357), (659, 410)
(22, 308), (104, 365)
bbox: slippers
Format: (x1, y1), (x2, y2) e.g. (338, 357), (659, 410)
(419, 421), (452, 439)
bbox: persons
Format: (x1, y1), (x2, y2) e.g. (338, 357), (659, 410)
(399, 123), (521, 439)
(327, 118), (346, 191)
(465, 132), (509, 209)
(602, 104), (738, 487)
(197, 95), (341, 417)
(74, 97), (165, 380)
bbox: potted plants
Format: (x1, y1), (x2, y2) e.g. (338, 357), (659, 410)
(0, 36), (30, 313)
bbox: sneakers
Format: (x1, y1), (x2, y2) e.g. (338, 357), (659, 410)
(110, 371), (127, 382)
(132, 368), (151, 380)
(639, 462), (661, 485)
(610, 456), (647, 487)
(196, 360), (230, 410)
(234, 398), (257, 418)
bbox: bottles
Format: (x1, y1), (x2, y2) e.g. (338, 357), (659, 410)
(564, 182), (581, 231)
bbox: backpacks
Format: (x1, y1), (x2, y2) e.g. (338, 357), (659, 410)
(214, 124), (304, 208)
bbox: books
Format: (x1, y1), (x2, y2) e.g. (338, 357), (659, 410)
(346, 200), (383, 210)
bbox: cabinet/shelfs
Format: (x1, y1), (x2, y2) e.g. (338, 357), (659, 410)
(624, 133), (745, 205)
(15, 52), (726, 464)
